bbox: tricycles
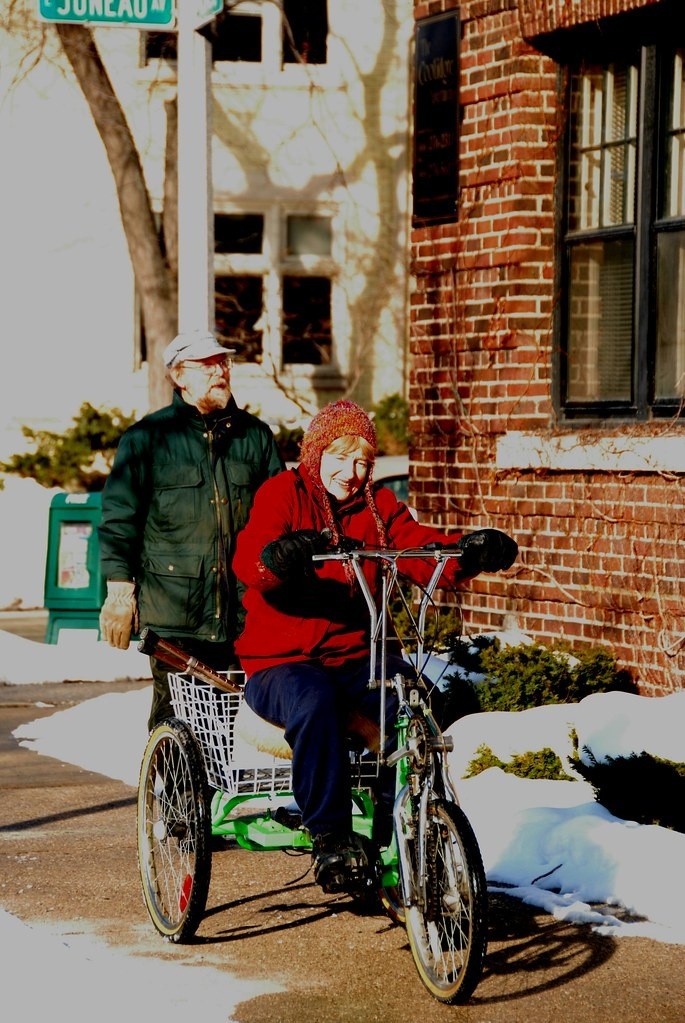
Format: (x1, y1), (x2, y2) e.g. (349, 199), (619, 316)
(135, 527), (490, 1007)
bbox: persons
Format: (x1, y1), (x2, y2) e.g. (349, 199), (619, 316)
(97, 330), (287, 851)
(232, 401), (518, 885)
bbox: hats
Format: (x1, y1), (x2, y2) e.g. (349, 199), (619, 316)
(302, 398), (392, 594)
(162, 330), (236, 369)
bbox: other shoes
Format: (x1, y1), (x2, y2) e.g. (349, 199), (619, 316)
(310, 834), (347, 885)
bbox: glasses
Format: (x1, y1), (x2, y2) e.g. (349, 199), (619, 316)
(177, 358), (233, 375)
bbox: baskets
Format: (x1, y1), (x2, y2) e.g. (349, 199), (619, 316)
(167, 670), (377, 796)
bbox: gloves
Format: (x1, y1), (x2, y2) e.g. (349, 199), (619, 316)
(99, 579), (138, 650)
(271, 529), (327, 575)
(456, 529), (519, 574)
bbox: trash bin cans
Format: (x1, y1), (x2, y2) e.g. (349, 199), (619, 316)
(41, 491), (141, 646)
